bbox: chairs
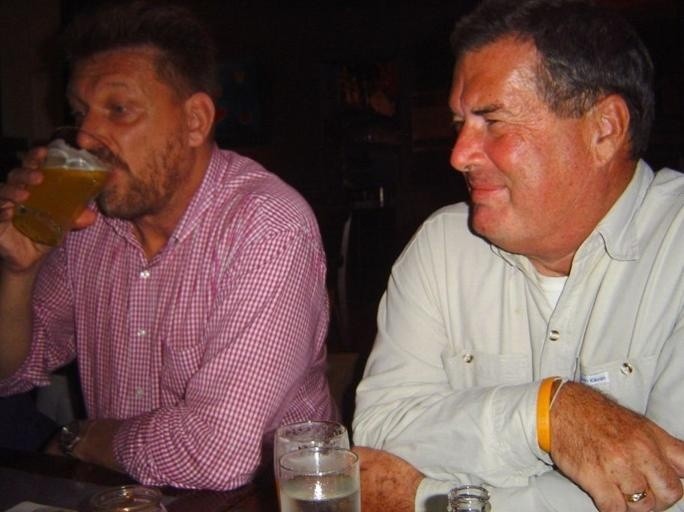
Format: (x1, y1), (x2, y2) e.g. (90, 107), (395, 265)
(315, 206), (363, 439)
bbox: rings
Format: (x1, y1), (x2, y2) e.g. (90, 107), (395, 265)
(622, 483), (650, 503)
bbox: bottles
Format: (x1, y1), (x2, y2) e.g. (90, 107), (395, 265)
(449, 485), (491, 511)
(89, 485), (166, 512)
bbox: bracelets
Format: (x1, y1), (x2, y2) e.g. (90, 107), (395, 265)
(535, 374), (561, 454)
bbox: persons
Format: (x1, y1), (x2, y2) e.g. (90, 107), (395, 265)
(345, 1), (684, 511)
(0, 1), (346, 493)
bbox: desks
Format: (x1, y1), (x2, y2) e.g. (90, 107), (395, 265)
(0, 466), (275, 512)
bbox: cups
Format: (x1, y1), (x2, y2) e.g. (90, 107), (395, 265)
(11, 127), (113, 247)
(276, 421), (351, 496)
(281, 448), (360, 512)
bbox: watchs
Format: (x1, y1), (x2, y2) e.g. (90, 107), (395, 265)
(58, 420), (81, 454)
